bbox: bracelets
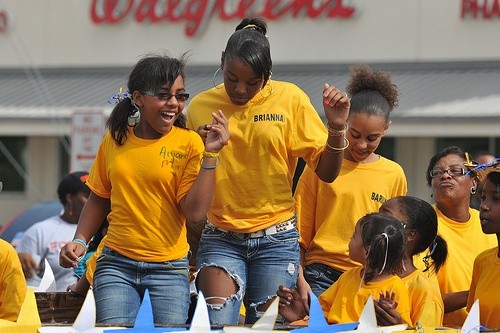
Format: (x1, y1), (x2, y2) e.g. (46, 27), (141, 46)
(72, 239), (88, 261)
(200, 150), (220, 169)
(325, 121), (349, 154)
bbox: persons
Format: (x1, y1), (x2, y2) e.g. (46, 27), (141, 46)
(0, 171), (109, 333)
(186, 17), (351, 327)
(59, 55), (230, 326)
(294, 63), (407, 298)
(411, 146), (498, 328)
(276, 196), (448, 328)
(317, 212), (412, 328)
(465, 170), (500, 329)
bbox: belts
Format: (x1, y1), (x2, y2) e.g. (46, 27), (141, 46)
(206, 217), (297, 238)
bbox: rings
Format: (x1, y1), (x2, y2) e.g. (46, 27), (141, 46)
(344, 93), (348, 97)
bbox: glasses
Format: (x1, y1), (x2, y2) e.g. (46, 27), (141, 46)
(141, 91), (189, 102)
(430, 168), (470, 176)
